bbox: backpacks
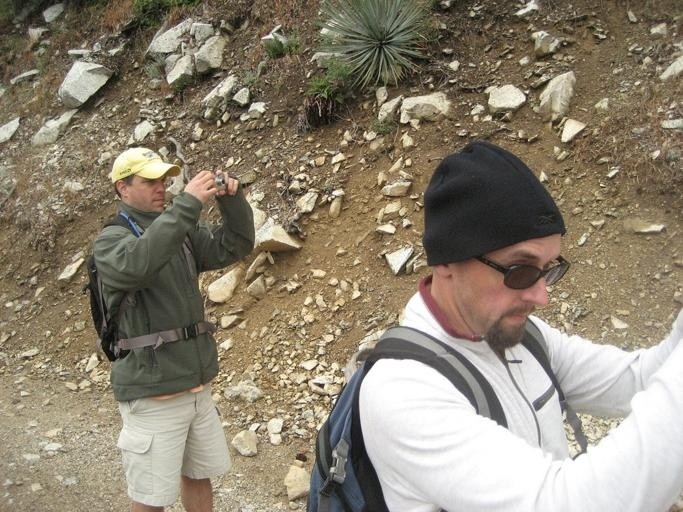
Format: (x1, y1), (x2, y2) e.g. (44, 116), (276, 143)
(86, 218), (144, 361)
(308, 316), (565, 510)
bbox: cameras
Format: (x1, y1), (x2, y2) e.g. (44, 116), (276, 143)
(213, 174), (229, 194)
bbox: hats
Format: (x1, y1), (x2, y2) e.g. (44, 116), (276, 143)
(421, 142), (565, 265)
(111, 149), (181, 184)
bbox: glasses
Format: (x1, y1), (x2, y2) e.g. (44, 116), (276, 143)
(477, 255), (570, 286)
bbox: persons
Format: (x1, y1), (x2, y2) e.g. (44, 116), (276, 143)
(358, 142), (683, 512)
(94, 147), (255, 512)
